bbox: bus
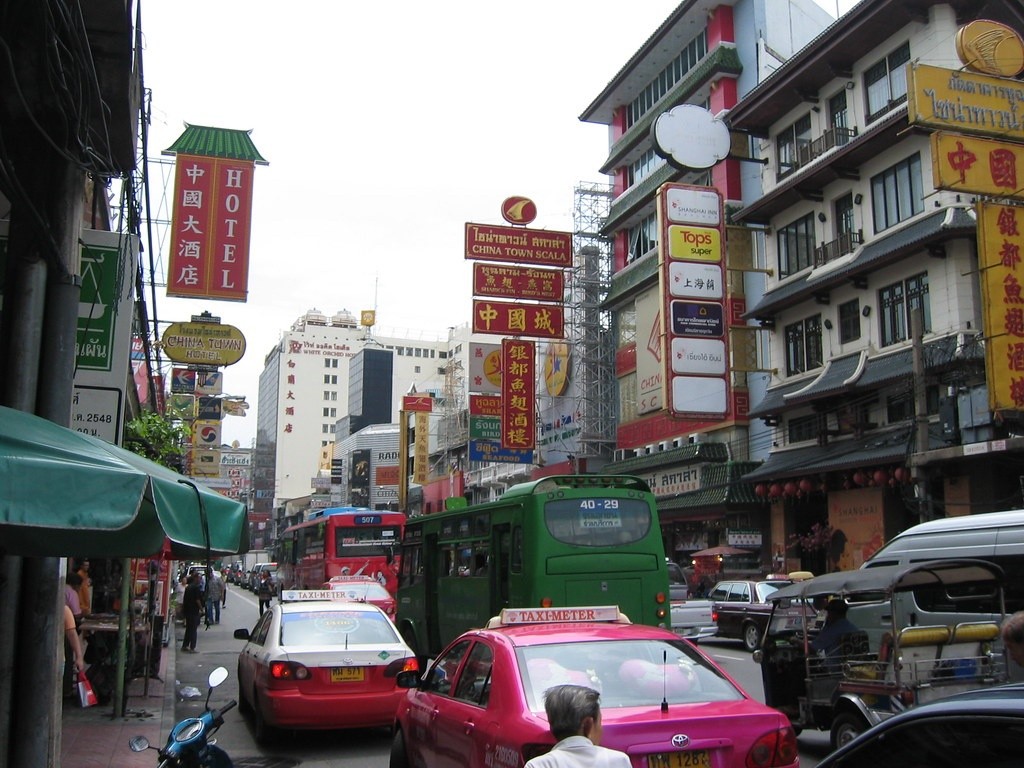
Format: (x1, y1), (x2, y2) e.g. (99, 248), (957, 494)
(271, 507), (407, 625)
(387, 475), (672, 659)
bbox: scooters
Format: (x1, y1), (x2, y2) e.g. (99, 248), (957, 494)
(753, 559), (1012, 751)
(129, 666), (238, 768)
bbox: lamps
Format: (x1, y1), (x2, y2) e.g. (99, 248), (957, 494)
(811, 106), (819, 112)
(845, 81), (855, 90)
(818, 211), (825, 223)
(855, 193), (862, 205)
(935, 200), (940, 207)
(956, 194), (960, 201)
(863, 305), (870, 317)
(824, 318), (833, 329)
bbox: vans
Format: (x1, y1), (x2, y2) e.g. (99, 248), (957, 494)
(846, 510), (1024, 682)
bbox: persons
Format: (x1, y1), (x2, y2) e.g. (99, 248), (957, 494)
(459, 554), (486, 577)
(1001, 611), (1024, 667)
(181, 573), (204, 653)
(61, 560), (92, 677)
(522, 685), (633, 768)
(781, 599), (859, 717)
(259, 570), (272, 617)
(175, 568), (227, 627)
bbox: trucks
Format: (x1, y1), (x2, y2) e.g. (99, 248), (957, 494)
(238, 550), (269, 589)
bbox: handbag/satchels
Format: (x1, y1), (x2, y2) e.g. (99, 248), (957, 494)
(76, 671), (97, 709)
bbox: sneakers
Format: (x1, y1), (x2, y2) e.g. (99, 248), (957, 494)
(182, 647), (190, 651)
(190, 649), (200, 653)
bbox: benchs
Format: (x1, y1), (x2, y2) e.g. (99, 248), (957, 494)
(845, 618), (995, 684)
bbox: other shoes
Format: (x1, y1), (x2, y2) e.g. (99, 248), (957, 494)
(209, 621), (214, 624)
(215, 621), (219, 624)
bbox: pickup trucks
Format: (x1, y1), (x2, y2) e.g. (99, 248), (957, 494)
(666, 599), (718, 646)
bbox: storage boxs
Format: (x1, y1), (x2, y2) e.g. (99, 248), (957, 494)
(940, 659), (977, 678)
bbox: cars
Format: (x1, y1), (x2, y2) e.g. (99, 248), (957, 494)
(666, 563), (693, 601)
(389, 605), (801, 768)
(234, 589), (422, 744)
(815, 682), (1024, 768)
(224, 560), (242, 586)
(246, 563), (262, 591)
(316, 574), (396, 626)
(706, 580), (829, 653)
(187, 566), (214, 584)
(253, 563), (278, 595)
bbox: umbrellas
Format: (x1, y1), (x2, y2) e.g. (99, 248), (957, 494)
(690, 546), (753, 556)
(0, 404), (251, 631)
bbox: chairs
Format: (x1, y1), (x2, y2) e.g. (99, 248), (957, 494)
(823, 629), (870, 674)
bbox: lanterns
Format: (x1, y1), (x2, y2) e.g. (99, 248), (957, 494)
(755, 465), (909, 506)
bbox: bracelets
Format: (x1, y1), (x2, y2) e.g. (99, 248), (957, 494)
(201, 610), (203, 611)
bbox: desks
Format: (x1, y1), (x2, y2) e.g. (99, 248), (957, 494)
(80, 617), (155, 697)
